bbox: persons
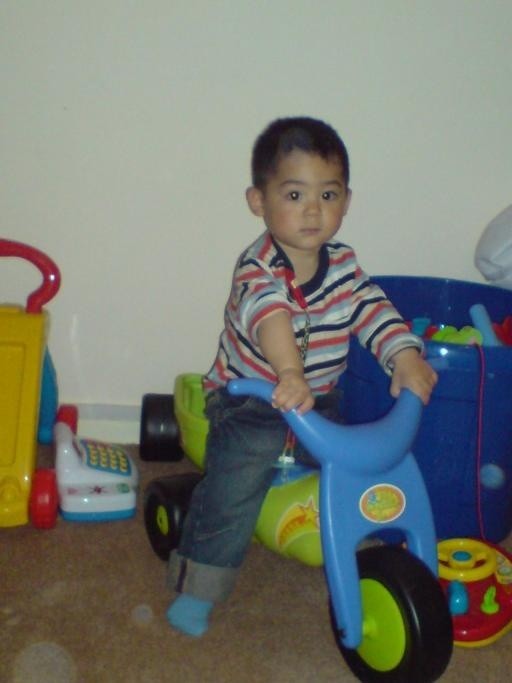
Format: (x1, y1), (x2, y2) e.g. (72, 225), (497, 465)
(166, 117), (441, 636)
